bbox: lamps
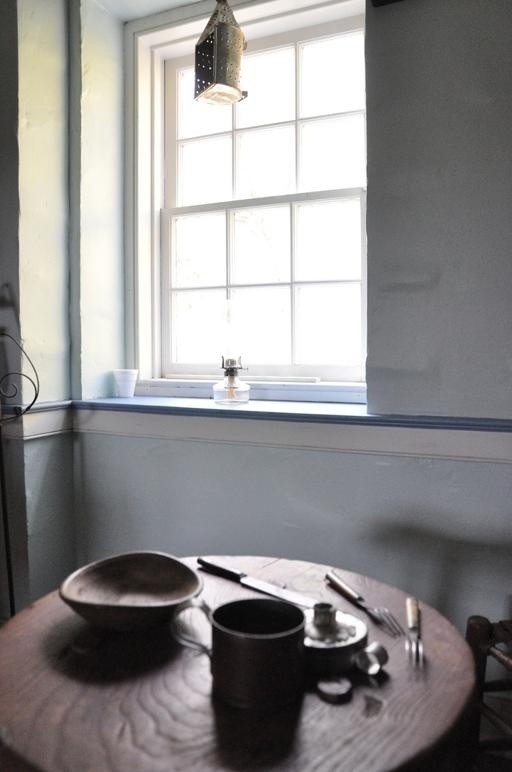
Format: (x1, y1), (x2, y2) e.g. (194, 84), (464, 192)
(195, 0), (249, 106)
(192, 299), (259, 405)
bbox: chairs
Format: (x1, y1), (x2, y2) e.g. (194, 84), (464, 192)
(452, 616), (512, 772)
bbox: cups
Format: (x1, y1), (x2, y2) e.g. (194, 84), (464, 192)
(168, 599), (307, 717)
(113, 369), (138, 398)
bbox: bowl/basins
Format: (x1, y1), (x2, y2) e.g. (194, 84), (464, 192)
(59, 551), (203, 632)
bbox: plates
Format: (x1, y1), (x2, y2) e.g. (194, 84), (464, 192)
(302, 609), (367, 650)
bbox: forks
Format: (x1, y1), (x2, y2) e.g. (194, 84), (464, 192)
(326, 568), (404, 638)
(404, 595), (425, 671)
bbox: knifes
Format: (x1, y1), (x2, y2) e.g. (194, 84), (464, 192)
(196, 557), (317, 608)
